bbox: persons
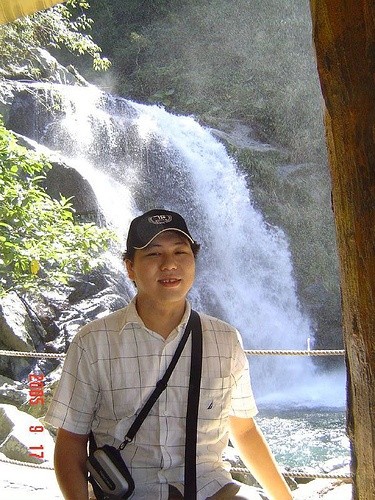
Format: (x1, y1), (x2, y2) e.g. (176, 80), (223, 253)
(43, 209), (296, 500)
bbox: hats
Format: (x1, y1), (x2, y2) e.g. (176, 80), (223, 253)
(126, 210), (196, 253)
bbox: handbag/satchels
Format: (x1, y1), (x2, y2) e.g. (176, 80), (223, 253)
(84, 444), (135, 499)
